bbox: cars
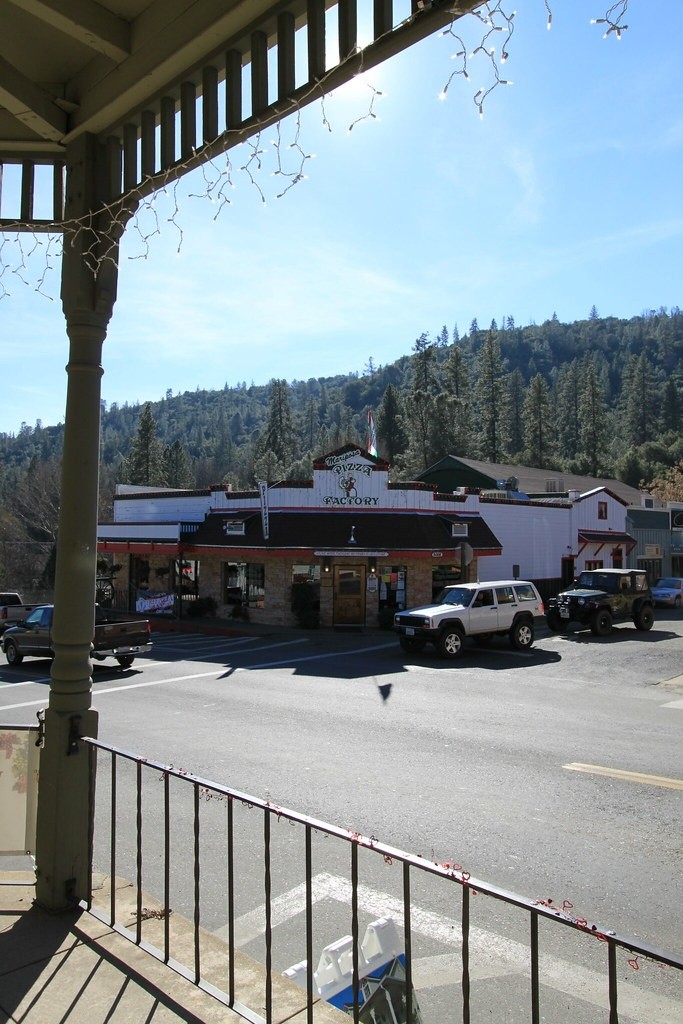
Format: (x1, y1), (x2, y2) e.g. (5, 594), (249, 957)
(650, 577), (683, 608)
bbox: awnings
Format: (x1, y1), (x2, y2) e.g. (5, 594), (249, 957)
(579, 533), (637, 558)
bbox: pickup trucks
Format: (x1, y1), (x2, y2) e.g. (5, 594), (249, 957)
(0, 603), (152, 666)
(0, 592), (52, 638)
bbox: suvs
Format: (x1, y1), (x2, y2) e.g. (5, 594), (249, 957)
(392, 581), (546, 661)
(547, 569), (656, 636)
